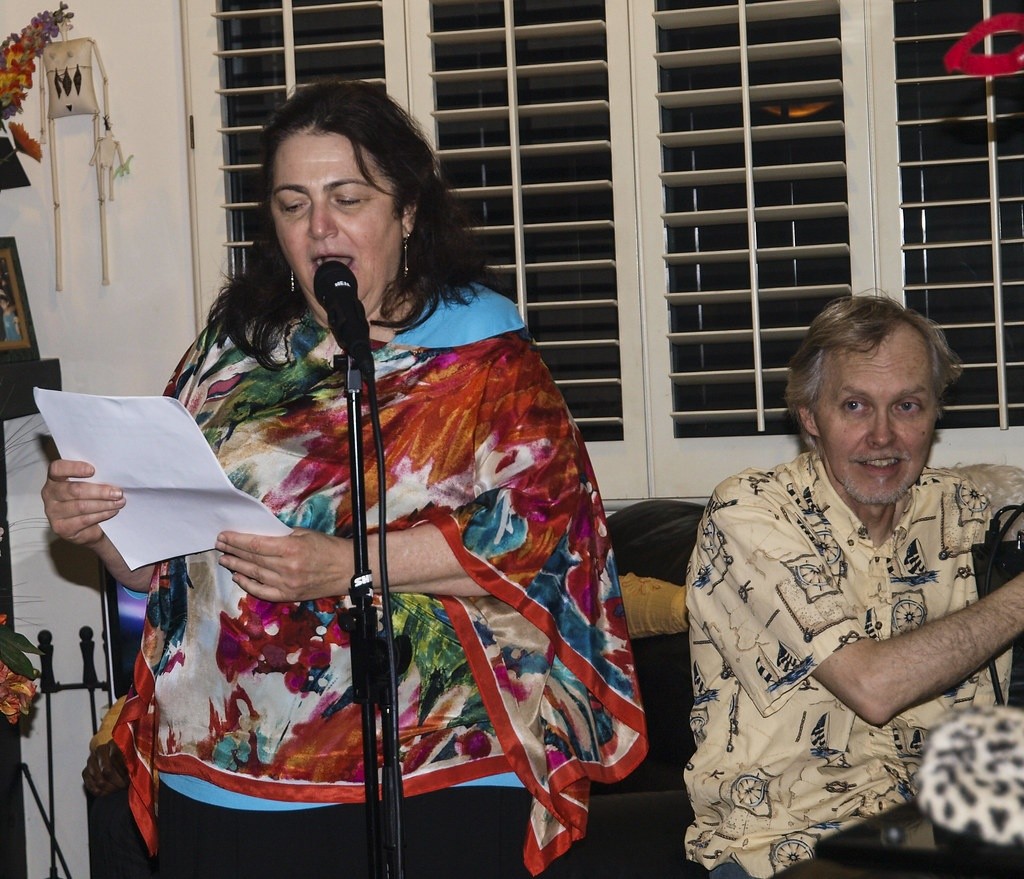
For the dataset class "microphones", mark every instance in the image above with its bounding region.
[314,260,374,373]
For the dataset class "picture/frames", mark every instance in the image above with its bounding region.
[0,236,40,364]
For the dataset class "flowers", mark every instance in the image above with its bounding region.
[0,614,46,724]
[0,3,74,163]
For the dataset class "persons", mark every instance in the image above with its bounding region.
[38,80,648,879]
[1,289,23,342]
[681,289,1024,878]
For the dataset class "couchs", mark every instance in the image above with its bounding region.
[566,500,705,879]
